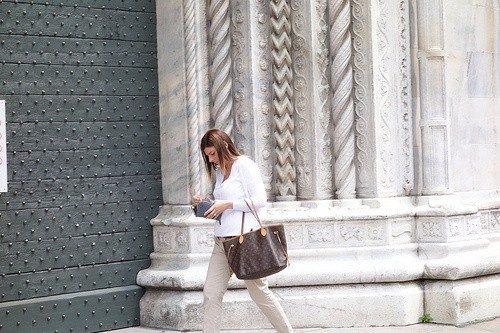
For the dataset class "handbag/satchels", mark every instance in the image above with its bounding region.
[222,198,291,279]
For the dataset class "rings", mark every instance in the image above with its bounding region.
[214,214,216,216]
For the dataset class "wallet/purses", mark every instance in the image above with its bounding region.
[191,196,221,225]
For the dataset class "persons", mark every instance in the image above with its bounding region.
[191,129,298,333]
